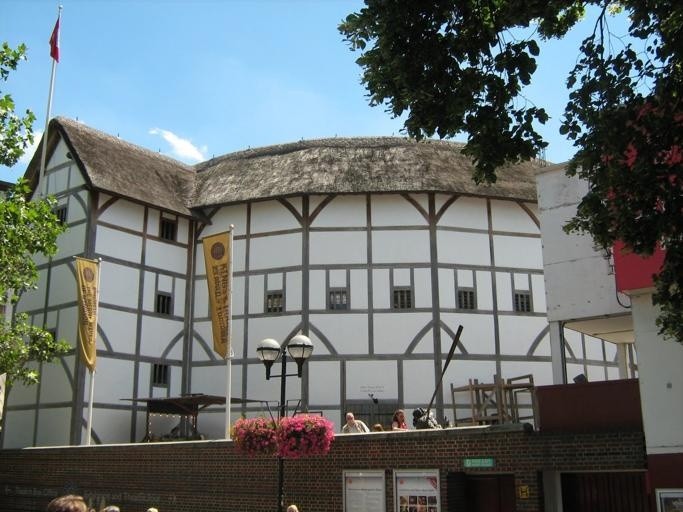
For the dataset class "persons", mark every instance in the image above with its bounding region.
[47,494,86,512]
[103,505,120,512]
[287,505,299,512]
[413,407,442,429]
[342,412,370,433]
[391,409,408,431]
[147,507,158,512]
[371,424,384,432]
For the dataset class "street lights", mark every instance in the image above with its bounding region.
[256,334,315,512]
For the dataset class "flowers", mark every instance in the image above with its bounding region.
[229,416,335,458]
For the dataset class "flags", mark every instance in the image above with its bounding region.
[48,17,60,63]
